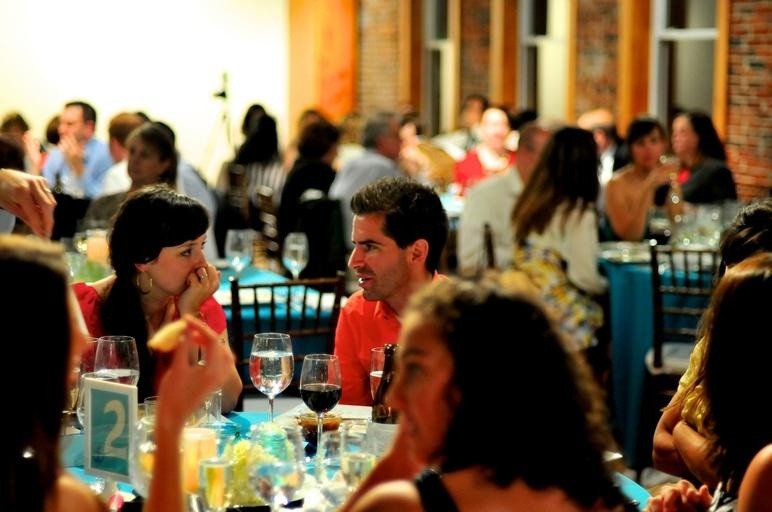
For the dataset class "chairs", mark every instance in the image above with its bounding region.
[229,272,347,411]
[643,239,720,448]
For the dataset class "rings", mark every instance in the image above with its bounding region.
[201,274,208,279]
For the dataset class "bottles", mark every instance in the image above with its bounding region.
[370,346,397,424]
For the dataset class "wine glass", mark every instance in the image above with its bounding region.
[300,353,345,466]
[249,330,294,428]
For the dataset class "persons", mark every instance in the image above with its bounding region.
[341,280,639,511]
[0,101,222,264]
[1,233,236,512]
[72,184,242,415]
[215,96,739,350]
[642,199,772,511]
[328,181,450,407]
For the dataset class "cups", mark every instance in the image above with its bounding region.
[636,199,749,254]
[280,232,309,279]
[182,389,370,512]
[47,171,86,202]
[659,156,681,180]
[59,229,110,285]
[128,416,191,503]
[58,336,158,438]
[370,346,387,400]
[225,227,255,276]
